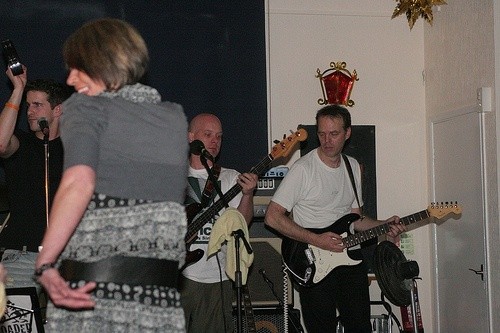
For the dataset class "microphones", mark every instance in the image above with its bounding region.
[37,116,53,137]
[260,269,269,281]
[189,140,213,161]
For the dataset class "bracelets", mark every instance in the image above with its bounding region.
[33,262,55,283]
[4,101,20,111]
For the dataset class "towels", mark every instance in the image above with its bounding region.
[206,207,255,286]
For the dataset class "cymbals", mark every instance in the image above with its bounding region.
[1,38,25,76]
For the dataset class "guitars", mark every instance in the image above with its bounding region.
[280,201,463,296]
[241,285,257,333]
[181,128,308,273]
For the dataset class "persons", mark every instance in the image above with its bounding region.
[265,106,406,333]
[176,112,259,333]
[0,17,189,333]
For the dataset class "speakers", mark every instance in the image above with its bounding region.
[231,218,301,333]
[297,125,377,221]
